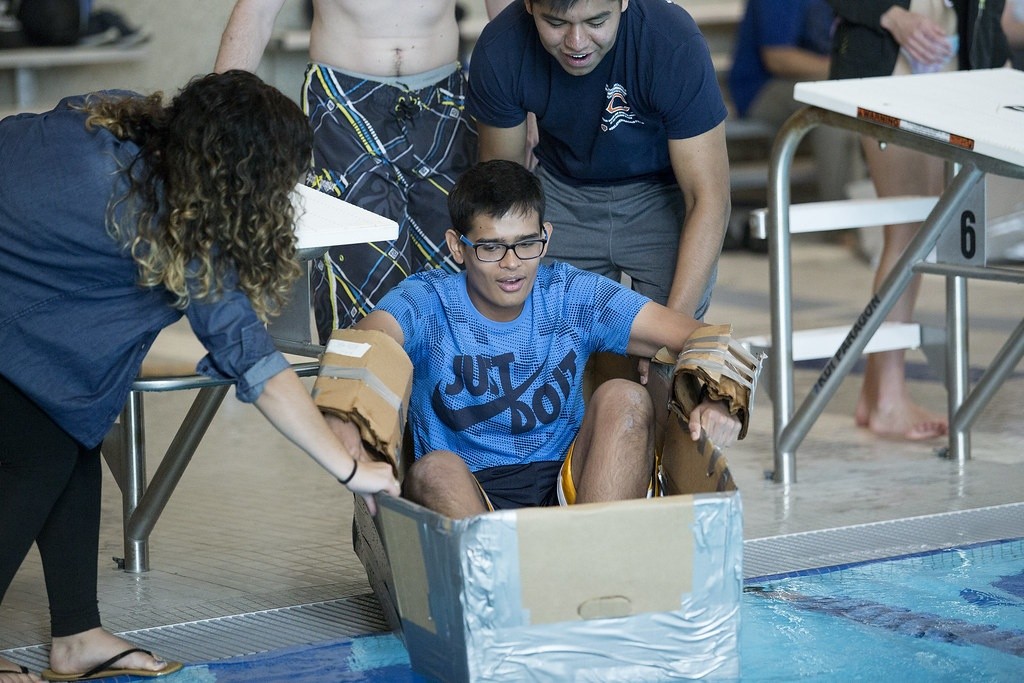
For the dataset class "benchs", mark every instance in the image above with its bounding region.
[0,41,150,116]
[267,0,873,244]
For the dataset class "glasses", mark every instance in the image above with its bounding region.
[451,221,549,262]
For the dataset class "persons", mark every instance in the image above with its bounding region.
[826,0,1024,443]
[729,0,881,247]
[323,159,745,522]
[1,69,402,682]
[212,2,517,347]
[465,0,733,387]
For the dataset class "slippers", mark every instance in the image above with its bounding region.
[0,664,35,683]
[40,647,183,680]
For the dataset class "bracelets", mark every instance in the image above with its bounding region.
[341,457,359,484]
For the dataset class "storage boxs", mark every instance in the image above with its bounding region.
[346,348,745,683]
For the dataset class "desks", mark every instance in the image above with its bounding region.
[101,181,402,580]
[750,61,1023,488]
